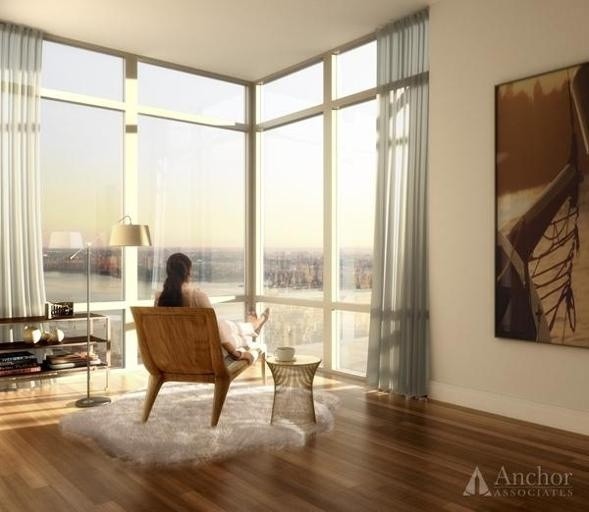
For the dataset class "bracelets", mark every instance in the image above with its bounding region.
[232,350,243,361]
[154,253,270,366]
[228,347,236,354]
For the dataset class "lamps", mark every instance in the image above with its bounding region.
[70,216,151,409]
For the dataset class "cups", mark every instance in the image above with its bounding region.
[274,348,295,361]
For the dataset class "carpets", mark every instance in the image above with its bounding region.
[60,381,339,477]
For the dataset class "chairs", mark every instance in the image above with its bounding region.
[128,304,266,427]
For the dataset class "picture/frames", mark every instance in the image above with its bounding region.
[494,62,589,349]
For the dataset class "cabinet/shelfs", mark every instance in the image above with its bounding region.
[1,314,115,401]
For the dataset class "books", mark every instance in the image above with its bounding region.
[0,351,42,377]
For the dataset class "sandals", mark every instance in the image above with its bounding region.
[247,306,270,336]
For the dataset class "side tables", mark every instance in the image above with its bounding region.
[264,358,319,425]
